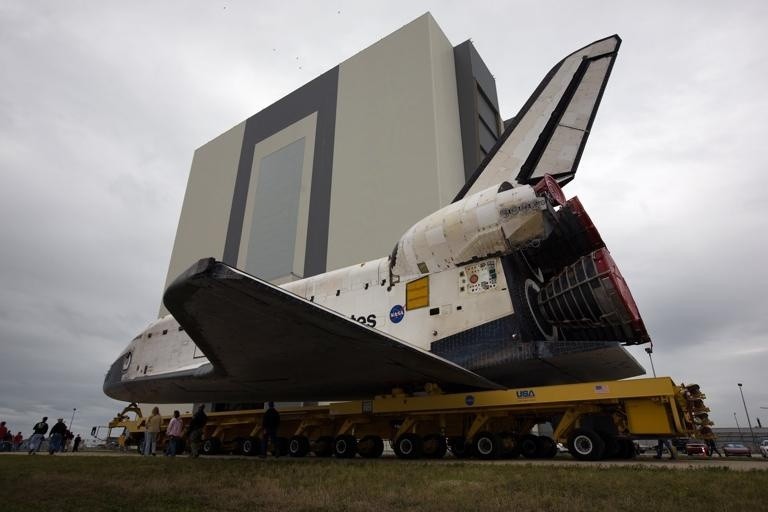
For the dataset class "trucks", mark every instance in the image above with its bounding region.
[106,376,715,460]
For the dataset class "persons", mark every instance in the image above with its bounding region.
[34,435,45,453]
[0,420,7,452]
[162,410,183,457]
[12,431,23,452]
[47,416,66,455]
[185,402,209,459]
[61,426,70,453]
[143,406,164,457]
[258,401,282,460]
[704,438,721,458]
[118,432,127,454]
[72,433,82,453]
[64,431,74,452]
[27,417,49,456]
[3,430,13,452]
[650,439,676,461]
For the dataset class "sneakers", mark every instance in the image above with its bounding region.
[652,452,677,460]
[27,448,56,455]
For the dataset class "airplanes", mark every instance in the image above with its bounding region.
[104,35,651,403]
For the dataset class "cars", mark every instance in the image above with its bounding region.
[686,444,707,455]
[760,440,768,457]
[725,442,751,458]
[556,443,569,453]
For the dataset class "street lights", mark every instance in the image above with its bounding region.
[738,383,757,450]
[645,347,657,378]
